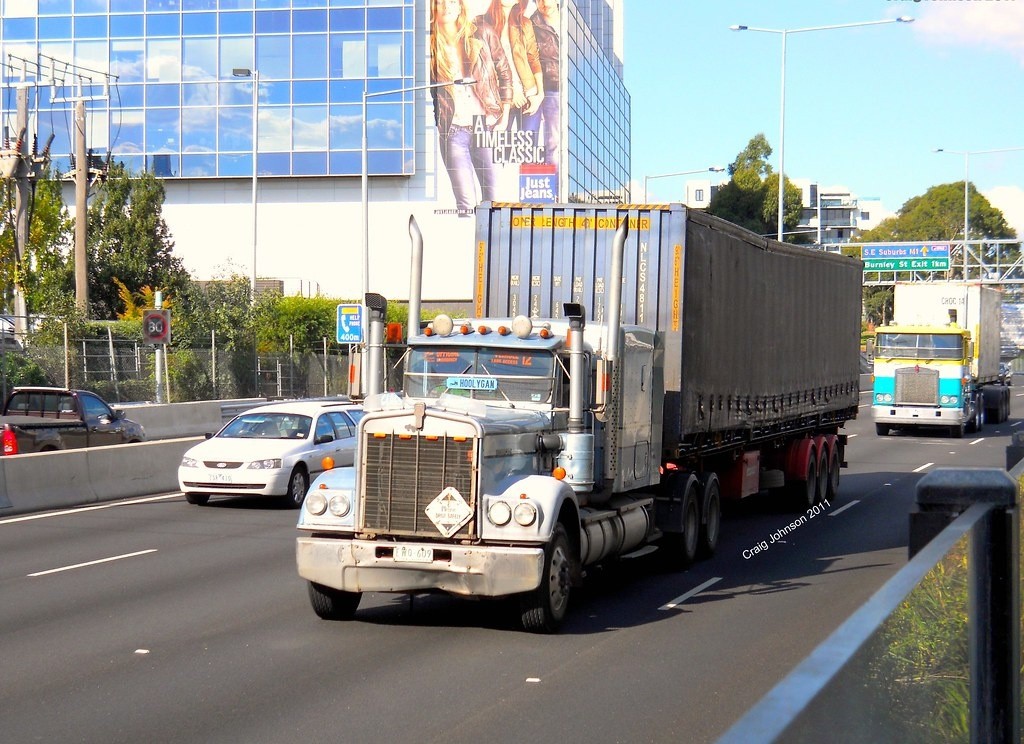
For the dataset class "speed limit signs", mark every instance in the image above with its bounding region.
[141,307,172,346]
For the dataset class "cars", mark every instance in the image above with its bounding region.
[176,395,371,511]
[0,313,39,357]
[994,361,1014,386]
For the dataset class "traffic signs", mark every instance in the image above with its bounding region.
[861,243,951,273]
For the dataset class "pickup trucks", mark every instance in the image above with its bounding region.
[0,385,149,456]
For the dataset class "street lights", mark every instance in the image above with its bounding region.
[359,75,481,397]
[933,146,1024,280]
[812,190,854,250]
[231,68,259,306]
[643,166,725,204]
[727,16,917,243]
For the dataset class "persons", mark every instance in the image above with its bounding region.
[430,360,498,400]
[429,0,560,214]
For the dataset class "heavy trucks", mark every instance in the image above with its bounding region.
[286,201,865,635]
[863,281,1011,438]
